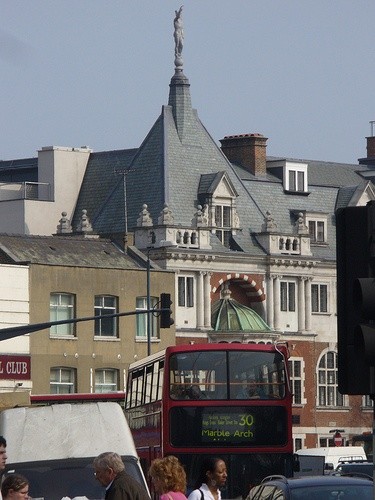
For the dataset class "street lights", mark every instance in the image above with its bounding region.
[147,241,174,356]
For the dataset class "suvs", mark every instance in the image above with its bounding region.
[244,459,375,500]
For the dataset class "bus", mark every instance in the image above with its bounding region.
[122,343,300,500]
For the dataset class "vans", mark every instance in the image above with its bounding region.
[0,401,152,500]
[293,446,367,476]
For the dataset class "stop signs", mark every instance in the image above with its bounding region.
[333,433,343,447]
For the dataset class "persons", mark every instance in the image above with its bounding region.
[149,456,188,500]
[93,453,151,500]
[0,436,30,500]
[184,385,206,400]
[235,380,259,398]
[173,4,185,58]
[187,456,228,500]
